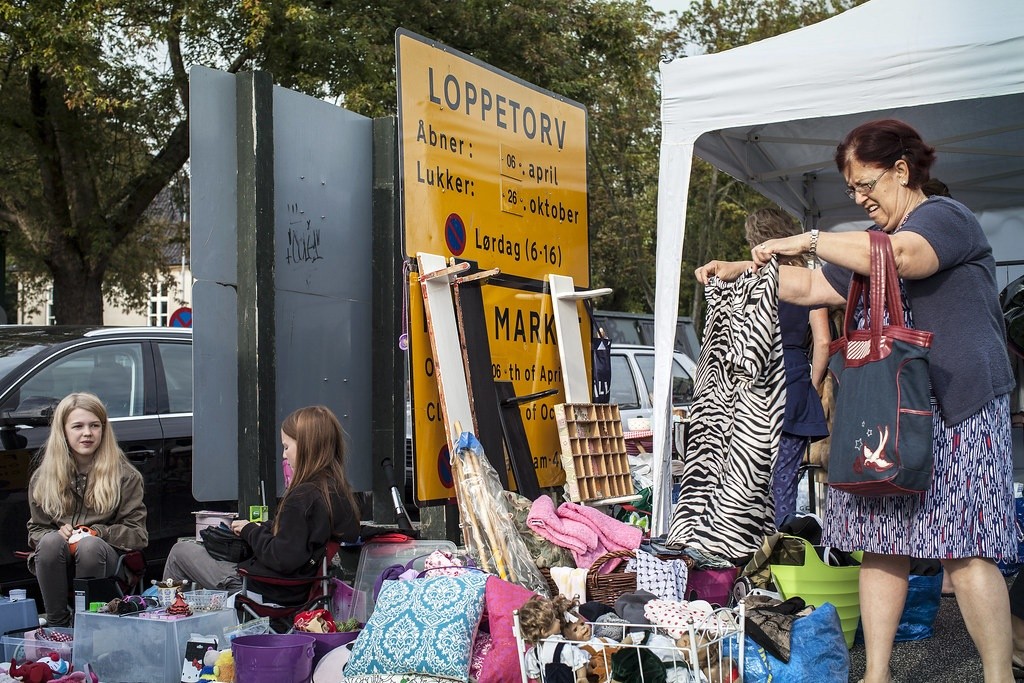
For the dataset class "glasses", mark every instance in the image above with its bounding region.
[845,160,901,201]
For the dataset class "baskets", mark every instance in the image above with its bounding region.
[587,550,639,604]
[185,590,229,613]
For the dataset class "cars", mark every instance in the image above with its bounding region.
[0,322,239,614]
[610,346,698,433]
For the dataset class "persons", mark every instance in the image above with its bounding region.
[519,593,739,683]
[694,118,1019,683]
[163,405,361,605]
[744,204,830,526]
[28,392,149,630]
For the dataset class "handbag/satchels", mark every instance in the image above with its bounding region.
[584,297,611,404]
[827,228,935,496]
[200,523,255,563]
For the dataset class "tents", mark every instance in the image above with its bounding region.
[651,0,1024,540]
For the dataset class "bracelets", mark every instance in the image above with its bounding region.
[810,229,818,256]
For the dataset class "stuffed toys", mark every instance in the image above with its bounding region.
[9,651,98,683]
[199,647,233,683]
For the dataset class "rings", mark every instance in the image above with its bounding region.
[760,243,766,249]
